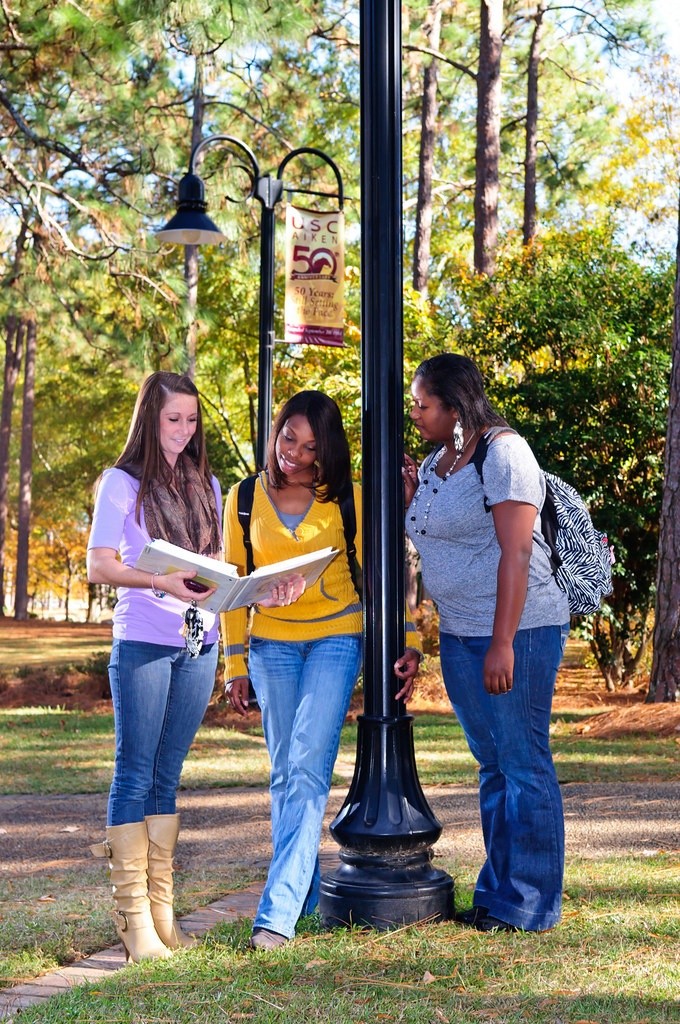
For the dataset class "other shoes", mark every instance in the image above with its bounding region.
[450,905,527,933]
[247,928,290,951]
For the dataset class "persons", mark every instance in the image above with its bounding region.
[224,389,425,950]
[401,353,570,933]
[86,373,304,963]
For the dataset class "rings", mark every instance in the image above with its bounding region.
[279,596,285,600]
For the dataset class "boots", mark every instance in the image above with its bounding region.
[88,821,176,965]
[144,813,203,950]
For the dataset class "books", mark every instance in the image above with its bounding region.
[134,539,340,614]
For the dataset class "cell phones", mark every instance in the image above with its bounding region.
[184,580,209,593]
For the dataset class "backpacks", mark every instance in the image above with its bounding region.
[472,432,616,616]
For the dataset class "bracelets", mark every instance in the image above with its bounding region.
[151,573,166,598]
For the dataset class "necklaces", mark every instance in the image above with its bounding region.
[411,432,475,535]
